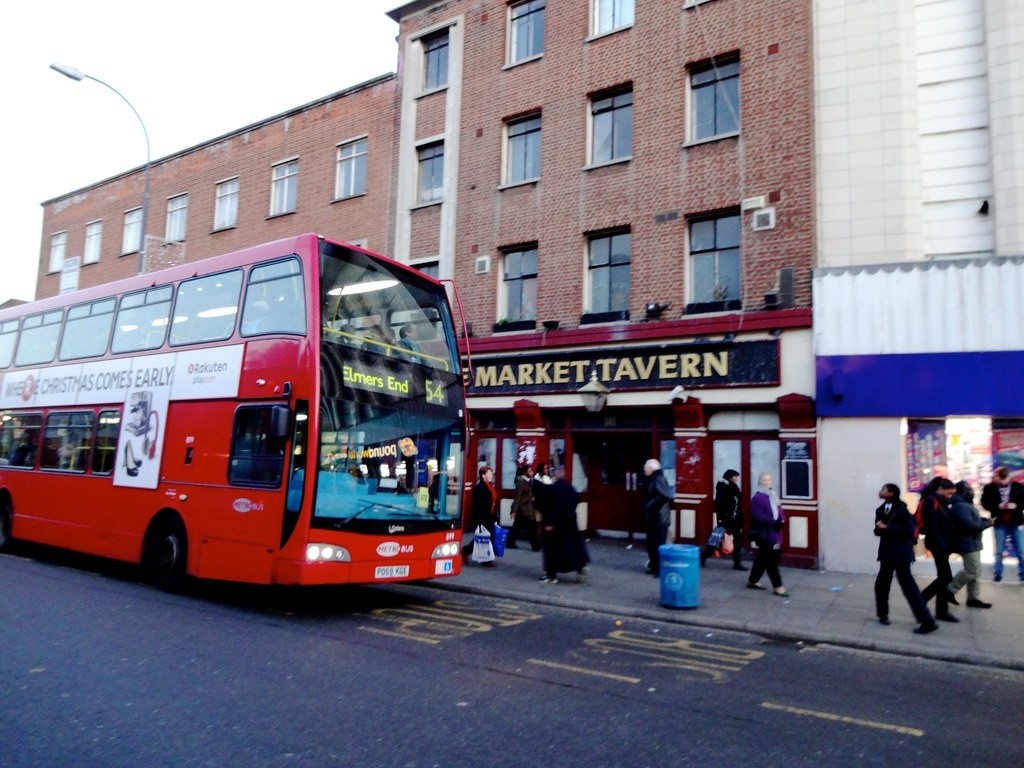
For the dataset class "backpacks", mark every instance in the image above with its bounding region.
[915,496,938,537]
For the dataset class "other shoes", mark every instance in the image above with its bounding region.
[936,612,959,622]
[482,562,496,567]
[540,576,559,584]
[645,568,658,577]
[699,552,705,567]
[733,564,748,571]
[773,590,789,597]
[967,600,991,608]
[994,574,1002,581]
[460,546,468,564]
[505,542,519,549]
[747,583,766,590]
[947,590,959,605]
[877,615,890,625]
[914,621,938,634]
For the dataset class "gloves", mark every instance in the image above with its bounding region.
[773,520,784,532]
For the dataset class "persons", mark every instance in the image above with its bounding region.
[643,459,670,576]
[504,463,591,584]
[746,472,790,596]
[337,324,356,345]
[367,325,394,354]
[12,428,40,466]
[872,484,940,634]
[948,482,994,608]
[982,467,1023,582]
[916,476,960,623]
[396,325,427,365]
[701,469,749,570]
[460,467,499,567]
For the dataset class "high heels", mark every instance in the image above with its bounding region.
[125,440,141,477]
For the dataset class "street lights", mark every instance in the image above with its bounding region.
[47,62,152,277]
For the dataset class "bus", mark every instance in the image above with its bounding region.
[0,232,474,597]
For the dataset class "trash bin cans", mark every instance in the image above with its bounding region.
[658,544,701,607]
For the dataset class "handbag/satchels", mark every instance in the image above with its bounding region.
[706,527,725,548]
[721,533,734,555]
[472,525,495,562]
[493,521,509,556]
